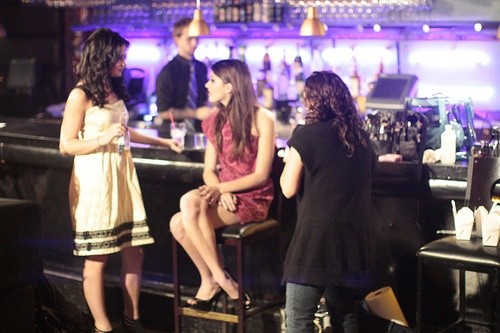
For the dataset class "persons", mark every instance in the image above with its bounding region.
[169,60,278,312]
[60,29,184,333]
[154,17,208,139]
[280,70,380,333]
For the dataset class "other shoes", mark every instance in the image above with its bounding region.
[123,313,146,333]
[92,327,117,333]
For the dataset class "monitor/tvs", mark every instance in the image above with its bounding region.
[365,73,418,111]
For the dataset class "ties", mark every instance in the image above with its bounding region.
[185,59,198,109]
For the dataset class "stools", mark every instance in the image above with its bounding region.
[172,217,282,333]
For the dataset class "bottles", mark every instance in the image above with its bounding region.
[440,124,456,166]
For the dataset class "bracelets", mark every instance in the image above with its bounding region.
[96,136,101,150]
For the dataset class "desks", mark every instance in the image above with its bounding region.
[0,196,44,303]
[416,220,500,333]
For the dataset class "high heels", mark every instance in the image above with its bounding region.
[189,288,224,311]
[227,295,247,315]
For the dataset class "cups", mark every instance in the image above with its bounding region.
[193,134,207,149]
[169,122,187,150]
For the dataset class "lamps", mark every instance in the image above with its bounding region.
[299,7,325,36]
[187,0,209,37]
